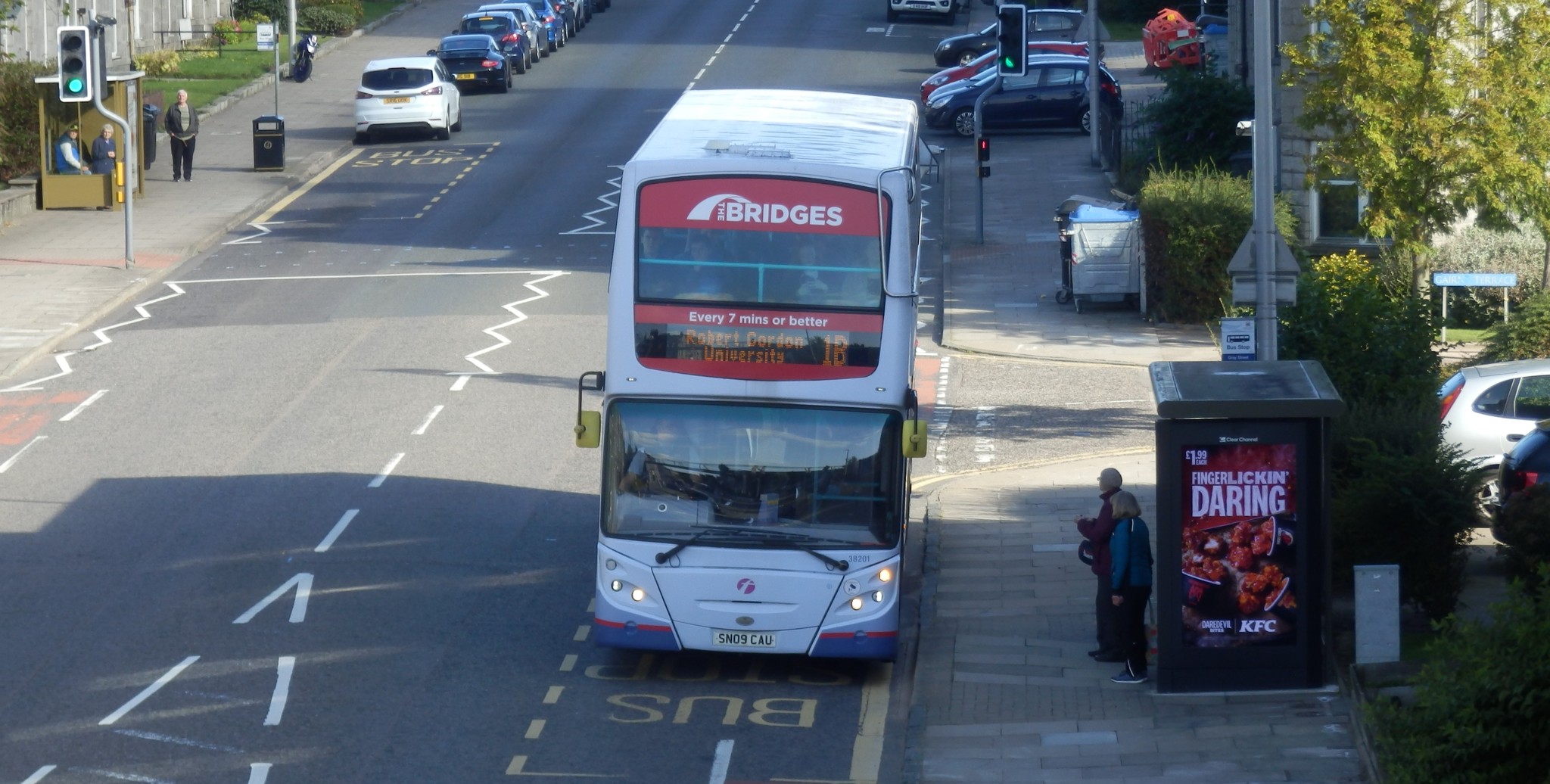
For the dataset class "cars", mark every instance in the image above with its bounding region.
[501,0,612,53]
[1491,418,1550,562]
[475,2,550,63]
[917,39,1106,105]
[353,57,462,146]
[924,53,1124,140]
[885,0,957,26]
[426,34,514,94]
[451,10,533,74]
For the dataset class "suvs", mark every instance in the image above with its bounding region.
[1435,358,1550,525]
[931,7,1089,69]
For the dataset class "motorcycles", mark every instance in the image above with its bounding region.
[292,34,319,83]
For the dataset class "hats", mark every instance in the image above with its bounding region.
[69,125,78,130]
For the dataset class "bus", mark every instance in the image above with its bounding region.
[570,87,928,668]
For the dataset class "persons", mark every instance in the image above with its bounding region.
[56,122,116,211]
[164,90,199,182]
[638,227,839,302]
[619,414,703,496]
[1108,491,1152,683]
[1073,468,1122,663]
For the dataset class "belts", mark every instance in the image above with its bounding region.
[183,140,187,147]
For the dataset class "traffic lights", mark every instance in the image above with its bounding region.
[997,3,1028,77]
[977,137,990,162]
[995,6,1027,79]
[56,25,91,102]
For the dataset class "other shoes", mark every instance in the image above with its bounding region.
[1141,671,1148,680]
[185,178,192,181]
[173,178,179,182]
[1088,650,1104,657]
[103,206,112,209]
[1095,652,1115,662]
[1111,672,1144,683]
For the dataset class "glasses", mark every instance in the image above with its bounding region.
[1097,477,1101,481]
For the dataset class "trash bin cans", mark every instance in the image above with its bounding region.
[253,116,285,172]
[143,112,154,170]
[143,104,161,162]
[1055,195,1126,305]
[1066,205,1147,314]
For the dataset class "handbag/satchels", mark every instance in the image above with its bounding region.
[1144,598,1159,665]
[1078,540,1095,565]
